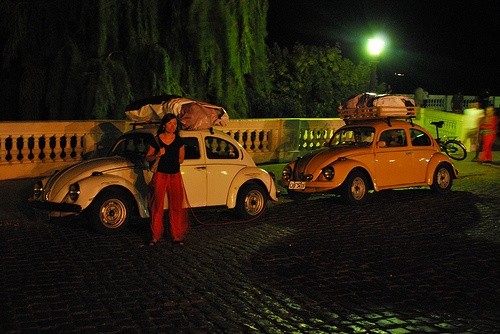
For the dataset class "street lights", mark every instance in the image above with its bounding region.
[366,37,386,93]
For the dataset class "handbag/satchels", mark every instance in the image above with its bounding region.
[142,170,154,192]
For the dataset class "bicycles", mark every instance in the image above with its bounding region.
[430,121,467,161]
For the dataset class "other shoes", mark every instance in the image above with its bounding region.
[178,240,185,247]
[149,239,158,247]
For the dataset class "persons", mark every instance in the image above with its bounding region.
[462,102,497,165]
[385,129,403,147]
[146,113,186,246]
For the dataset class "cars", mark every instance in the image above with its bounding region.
[277,116,459,207]
[27,121,281,236]
